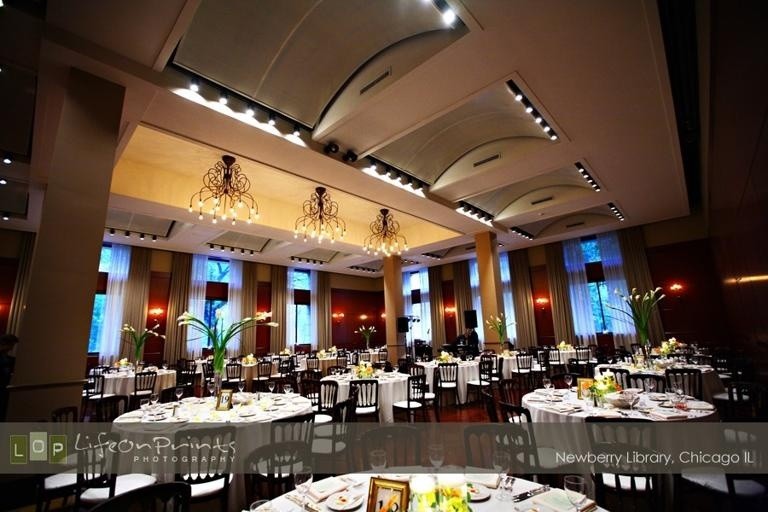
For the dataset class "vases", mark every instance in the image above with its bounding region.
[366,336,370,349]
[213,351,223,396]
[499,336,505,354]
[134,348,140,374]
[638,329,647,346]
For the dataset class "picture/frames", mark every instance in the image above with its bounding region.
[577,378,593,400]
[366,476,410,512]
[634,355,645,368]
[216,390,233,411]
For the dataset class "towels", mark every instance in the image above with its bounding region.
[464,466,503,488]
[551,403,576,414]
[650,408,689,422]
[534,486,587,512]
[308,476,346,500]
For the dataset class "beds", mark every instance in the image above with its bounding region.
[250,465,611,512]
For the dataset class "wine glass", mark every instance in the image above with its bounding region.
[564,476,589,512]
[624,391,639,419]
[645,377,656,399]
[284,385,290,398]
[544,379,551,397]
[286,388,293,401]
[371,450,386,478]
[466,355,473,364]
[268,382,275,395]
[492,450,512,501]
[607,356,613,366]
[140,398,149,421]
[294,466,313,512]
[580,389,592,413]
[162,362,168,370]
[393,365,400,375]
[207,383,215,398]
[428,443,444,474]
[332,367,346,378]
[249,500,272,512]
[150,391,159,416]
[564,375,572,396]
[176,388,182,406]
[381,364,385,370]
[417,356,420,363]
[238,382,244,395]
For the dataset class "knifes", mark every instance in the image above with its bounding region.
[284,494,318,512]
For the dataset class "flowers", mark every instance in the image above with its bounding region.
[351,363,373,379]
[654,337,679,355]
[115,357,129,367]
[328,345,337,353]
[245,353,253,364]
[280,347,290,355]
[415,483,474,512]
[117,323,166,359]
[353,325,377,340]
[583,376,622,399]
[178,308,279,372]
[591,286,665,343]
[436,351,453,364]
[317,349,326,358]
[485,312,517,344]
[556,340,569,350]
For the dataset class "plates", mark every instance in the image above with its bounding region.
[274,401,287,404]
[264,406,278,411]
[163,403,177,409]
[147,415,166,420]
[148,410,165,415]
[326,493,363,511]
[462,482,490,500]
[238,411,257,417]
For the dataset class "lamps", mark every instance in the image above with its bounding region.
[363,208,408,258]
[294,186,346,244]
[188,154,259,226]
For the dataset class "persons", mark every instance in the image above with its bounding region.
[0,331,18,416]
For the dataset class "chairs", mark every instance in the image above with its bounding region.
[312,399,353,471]
[94,367,109,375]
[244,440,308,510]
[682,421,768,512]
[438,362,461,409]
[630,343,640,354]
[350,379,379,423]
[466,360,493,410]
[327,366,346,376]
[481,388,499,426]
[510,353,532,390]
[301,370,321,393]
[599,367,629,388]
[392,374,426,424]
[712,365,760,416]
[588,344,598,366]
[360,352,370,362]
[626,374,666,394]
[546,348,565,379]
[584,416,657,454]
[298,378,320,413]
[337,355,347,367]
[567,347,589,377]
[409,364,425,394]
[345,424,421,473]
[251,361,272,393]
[270,412,315,473]
[81,375,116,424]
[463,422,530,482]
[156,422,234,512]
[727,383,768,423]
[202,363,214,397]
[272,358,281,364]
[665,368,703,402]
[128,371,157,412]
[161,385,187,405]
[498,401,573,487]
[90,481,191,512]
[536,348,546,368]
[306,357,319,370]
[182,360,195,376]
[379,351,387,361]
[94,395,128,439]
[480,354,504,398]
[304,380,342,428]
[408,367,441,423]
[592,440,674,512]
[50,406,78,433]
[41,431,120,512]
[222,363,242,391]
